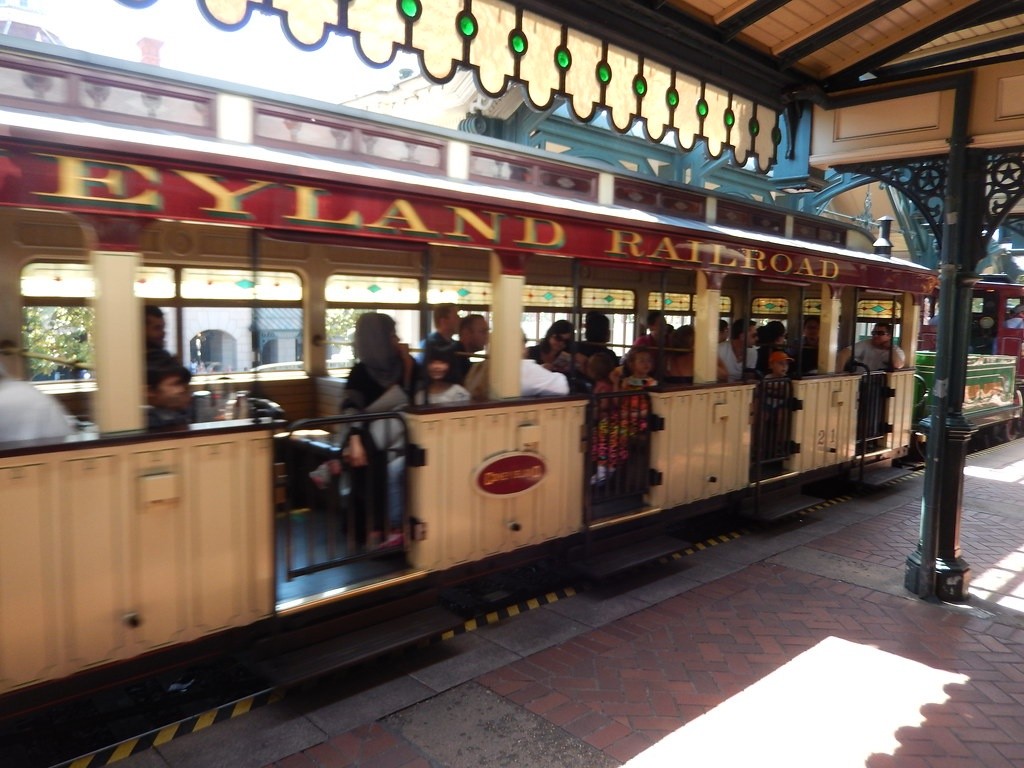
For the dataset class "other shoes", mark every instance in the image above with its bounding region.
[308,460,335,491]
[381,529,404,547]
[589,476,608,491]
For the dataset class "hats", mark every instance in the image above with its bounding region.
[768,350,794,363]
[1009,304,1024,313]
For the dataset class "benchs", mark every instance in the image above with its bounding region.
[277,423,344,456]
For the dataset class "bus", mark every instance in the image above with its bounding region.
[0,37,1024,693]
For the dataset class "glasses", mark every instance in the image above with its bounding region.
[550,334,570,343]
[871,331,888,336]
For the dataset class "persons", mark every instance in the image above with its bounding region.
[992,305,1024,355]
[1,298,905,550]
